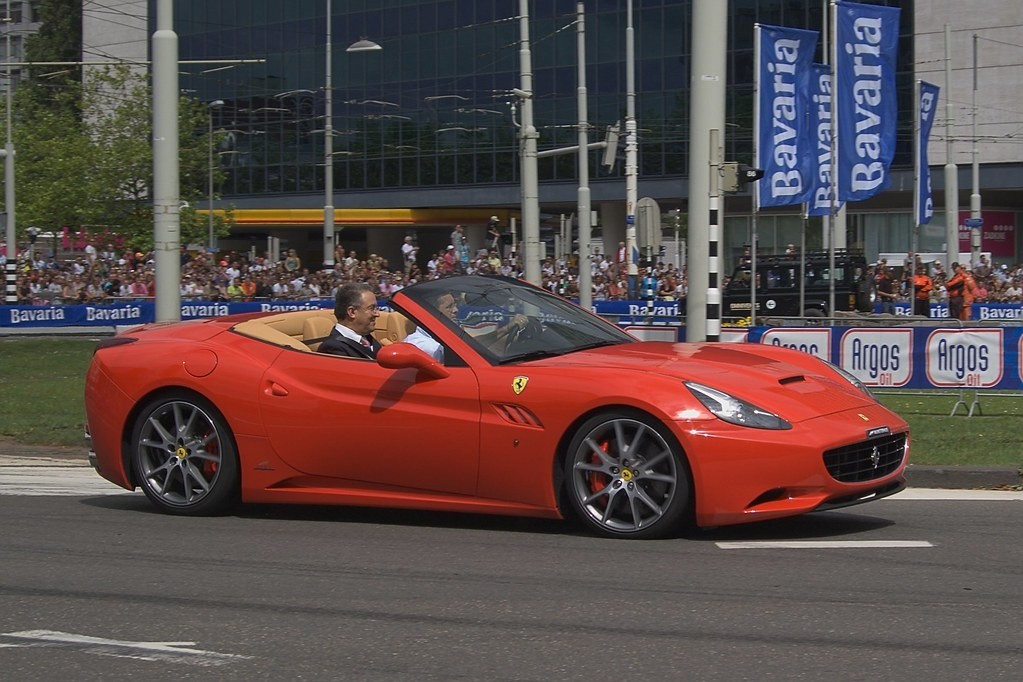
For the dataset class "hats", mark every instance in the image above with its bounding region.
[951,262,958,268]
[1000,264,1008,269]
[447,245,454,249]
[491,216,500,221]
[135,252,144,257]
[220,261,228,266]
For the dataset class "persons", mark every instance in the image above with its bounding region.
[2,216,1023,325]
[402,291,529,367]
[312,283,383,362]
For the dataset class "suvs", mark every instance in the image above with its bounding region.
[719,247,875,324]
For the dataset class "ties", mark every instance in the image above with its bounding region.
[360,338,371,349]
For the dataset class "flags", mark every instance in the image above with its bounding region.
[836,0,900,202]
[801,63,846,219]
[919,79,941,224]
[759,23,820,208]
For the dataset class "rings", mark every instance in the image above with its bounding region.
[520,320,523,323]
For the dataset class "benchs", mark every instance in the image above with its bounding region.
[254,310,342,346]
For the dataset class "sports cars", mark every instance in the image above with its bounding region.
[83,272,912,540]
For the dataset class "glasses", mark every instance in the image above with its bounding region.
[353,305,381,311]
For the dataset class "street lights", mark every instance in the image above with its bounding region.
[207,101,225,265]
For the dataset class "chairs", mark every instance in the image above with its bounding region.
[303,317,335,352]
[379,311,417,345]
